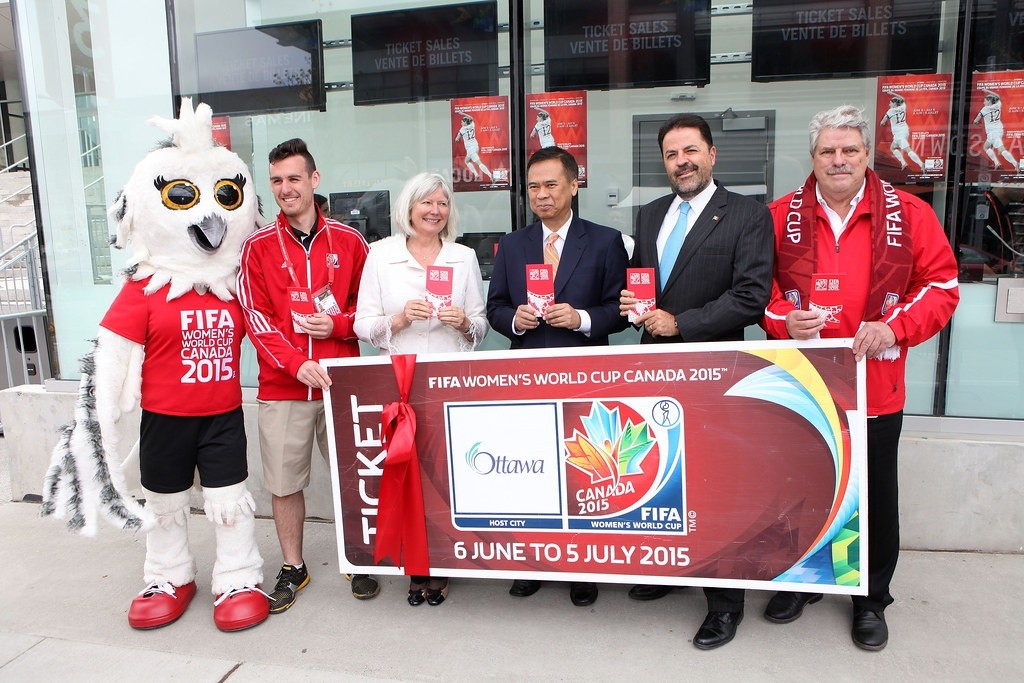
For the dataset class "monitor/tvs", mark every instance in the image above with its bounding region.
[971,0,1024,72]
[330,190,391,244]
[751,0,942,82]
[193,19,326,118]
[351,0,500,107]
[956,181,1024,283]
[462,232,507,280]
[544,0,712,92]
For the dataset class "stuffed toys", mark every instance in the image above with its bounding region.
[39,96,271,633]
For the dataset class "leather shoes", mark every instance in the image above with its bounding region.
[693,607,745,649]
[764,591,824,623]
[629,585,672,600]
[569,582,598,605]
[852,602,889,650]
[508,579,541,597]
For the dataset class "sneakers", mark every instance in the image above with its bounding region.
[267,559,310,613]
[345,574,380,599]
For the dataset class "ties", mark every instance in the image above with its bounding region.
[544,233,559,283]
[659,202,691,293]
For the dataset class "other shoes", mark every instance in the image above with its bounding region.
[1015,163,1022,174]
[922,163,927,175]
[901,162,908,172]
[426,577,449,605]
[994,163,1002,170]
[473,175,480,182]
[408,576,427,606]
[490,175,495,184]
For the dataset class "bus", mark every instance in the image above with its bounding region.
[892,181,1015,274]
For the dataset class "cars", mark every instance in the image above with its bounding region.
[958,245,996,283]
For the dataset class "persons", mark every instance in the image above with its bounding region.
[630,112,778,652]
[973,85,1020,173]
[760,105,962,652]
[880,91,927,176]
[232,138,379,613]
[352,171,491,606]
[485,145,633,606]
[529,106,555,148]
[455,109,494,183]
